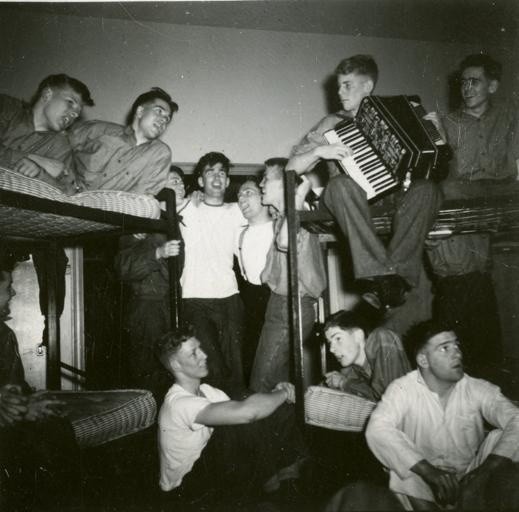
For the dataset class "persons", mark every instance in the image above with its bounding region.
[286,53,519,309]
[0,256,66,512]
[364,325,519,511]
[0,75,177,195]
[323,311,412,511]
[157,332,295,511]
[103,149,325,490]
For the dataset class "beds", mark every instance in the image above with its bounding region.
[1,165,184,452]
[278,170,517,464]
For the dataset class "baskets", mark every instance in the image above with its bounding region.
[304,384,376,434]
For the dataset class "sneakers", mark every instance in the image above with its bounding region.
[360,289,382,317]
[377,275,410,320]
